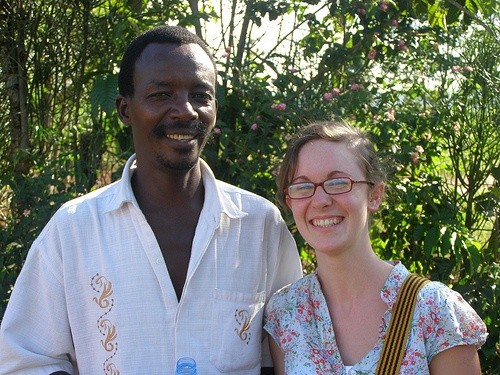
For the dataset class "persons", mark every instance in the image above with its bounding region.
[261,120,490,375]
[0,27,305,375]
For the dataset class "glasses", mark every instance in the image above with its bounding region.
[284,177,374,200]
